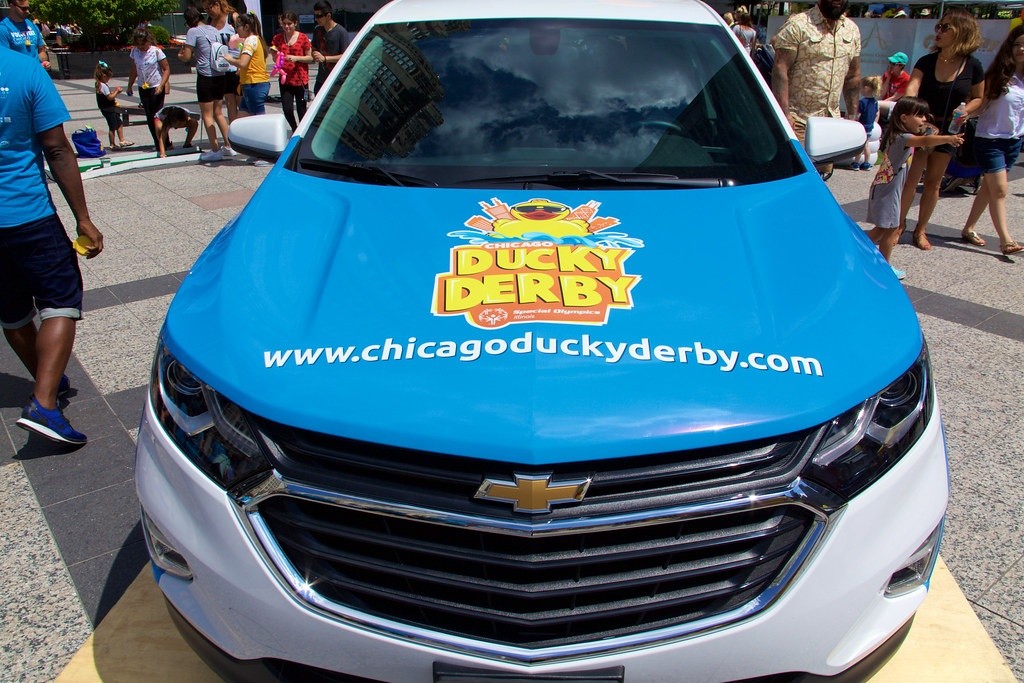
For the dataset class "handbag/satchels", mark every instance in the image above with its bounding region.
[72,127,106,158]
[156,49,170,94]
[304,90,315,101]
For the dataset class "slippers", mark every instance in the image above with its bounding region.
[1001,241,1024,255]
[962,231,985,246]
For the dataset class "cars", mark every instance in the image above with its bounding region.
[134,0,952,683]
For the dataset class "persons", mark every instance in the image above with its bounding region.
[722,0,1024,280]
[33,15,70,80]
[310,0,350,98]
[126,28,174,152]
[153,105,199,159]
[270,11,313,133]
[0,0,47,70]
[178,0,270,167]
[137,18,149,30]
[0,46,104,445]
[94,61,135,150]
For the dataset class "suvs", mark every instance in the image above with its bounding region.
[44,32,57,45]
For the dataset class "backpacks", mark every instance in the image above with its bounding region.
[194,27,231,72]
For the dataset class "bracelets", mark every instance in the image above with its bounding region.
[844,113,862,119]
[324,56,326,63]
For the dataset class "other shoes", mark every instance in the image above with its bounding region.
[166,141,174,150]
[847,162,871,170]
[201,149,221,161]
[245,157,274,167]
[182,143,193,148]
[889,263,906,280]
[152,146,156,150]
[221,145,237,156]
[109,145,124,151]
[119,140,134,147]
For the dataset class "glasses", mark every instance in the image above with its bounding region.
[16,5,28,12]
[934,24,953,32]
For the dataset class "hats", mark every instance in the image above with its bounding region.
[892,10,907,18]
[920,9,931,15]
[888,52,908,65]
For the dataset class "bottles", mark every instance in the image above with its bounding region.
[948,102,968,135]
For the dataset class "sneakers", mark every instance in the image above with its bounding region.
[56,374,71,395]
[15,397,88,445]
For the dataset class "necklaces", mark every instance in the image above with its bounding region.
[1015,71,1024,74]
[939,54,959,64]
